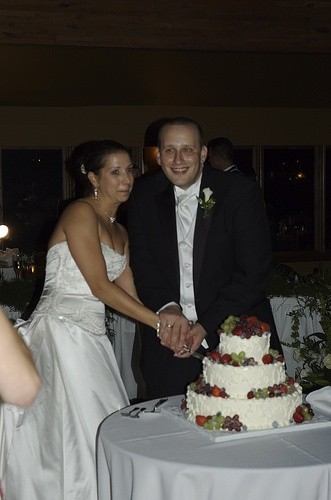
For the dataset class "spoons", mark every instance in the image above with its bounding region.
[121,406,140,416]
[144,398,168,413]
[129,407,146,418]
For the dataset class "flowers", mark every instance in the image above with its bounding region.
[267,265,331,393]
[196,187,217,220]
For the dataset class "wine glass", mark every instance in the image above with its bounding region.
[14,254,21,281]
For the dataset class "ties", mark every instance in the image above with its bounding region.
[177,196,193,238]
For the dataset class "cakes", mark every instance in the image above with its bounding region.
[182,315,315,431]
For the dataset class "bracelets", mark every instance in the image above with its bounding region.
[157,320,160,337]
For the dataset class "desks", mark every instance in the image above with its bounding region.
[95,394,330,500]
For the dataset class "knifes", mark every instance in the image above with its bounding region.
[182,345,204,360]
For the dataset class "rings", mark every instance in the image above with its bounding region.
[167,324,173,328]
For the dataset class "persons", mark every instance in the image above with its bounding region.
[122,117,271,400]
[0,139,191,500]
[207,137,242,173]
